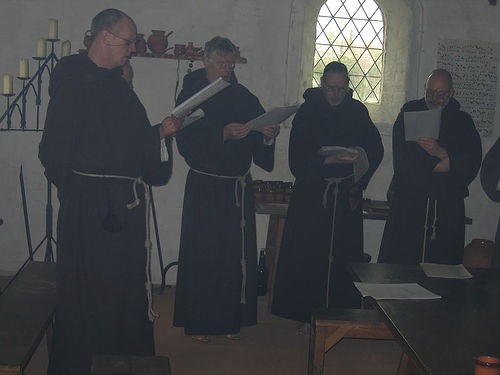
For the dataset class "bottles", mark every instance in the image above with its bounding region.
[256,250,269,297]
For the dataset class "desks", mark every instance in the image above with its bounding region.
[252,188,473,311]
[347,260,499,375]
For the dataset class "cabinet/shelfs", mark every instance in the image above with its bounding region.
[80,46,248,63]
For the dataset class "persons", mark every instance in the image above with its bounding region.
[34,7,182,374]
[173,34,282,345]
[271,59,385,325]
[377,67,483,267]
[479,127,499,274]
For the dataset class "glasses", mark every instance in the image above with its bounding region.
[323,82,348,93]
[108,31,138,46]
[207,59,236,69]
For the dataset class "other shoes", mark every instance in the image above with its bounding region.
[224,333,242,340]
[191,334,211,344]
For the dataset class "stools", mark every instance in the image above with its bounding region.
[308,308,400,375]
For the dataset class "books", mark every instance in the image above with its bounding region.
[169,76,233,119]
[352,280,442,299]
[417,260,473,280]
[400,106,445,145]
[244,97,306,137]
[317,144,371,187]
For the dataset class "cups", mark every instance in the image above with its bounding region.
[254,180,292,202]
[174,44,185,56]
[362,197,371,210]
[472,355,500,375]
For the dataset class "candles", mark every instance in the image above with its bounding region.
[36,38,47,57]
[48,16,58,39]
[2,75,13,94]
[62,40,71,56]
[19,58,30,77]
